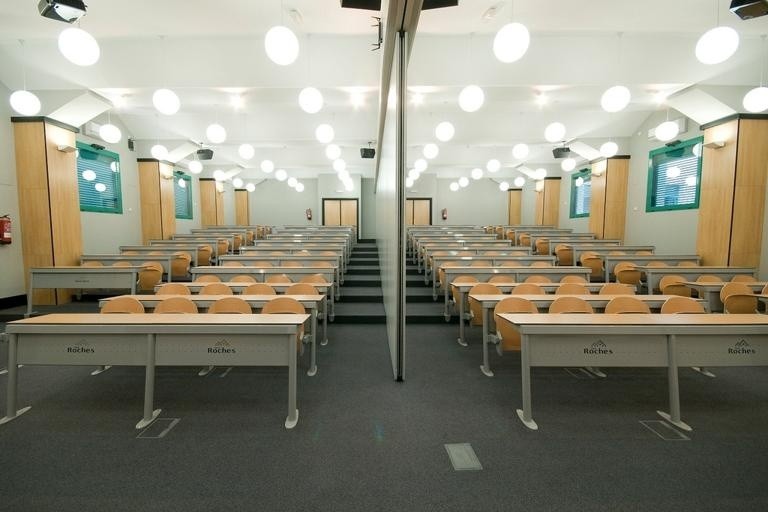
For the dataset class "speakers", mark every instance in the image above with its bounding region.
[553,148,572,159]
[197,150,213,160]
[38,0,86,24]
[361,148,375,158]
[729,0,768,21]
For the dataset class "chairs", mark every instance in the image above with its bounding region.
[468,294,768,432]
[0,225,357,431]
[403,223,768,347]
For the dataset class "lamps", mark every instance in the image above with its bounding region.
[264,24,300,66]
[10,87,357,197]
[492,23,531,63]
[694,26,741,66]
[57,28,101,69]
[405,84,767,194]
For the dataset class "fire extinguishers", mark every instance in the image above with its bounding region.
[306,208,311,220]
[0,214,12,244]
[442,208,447,220]
